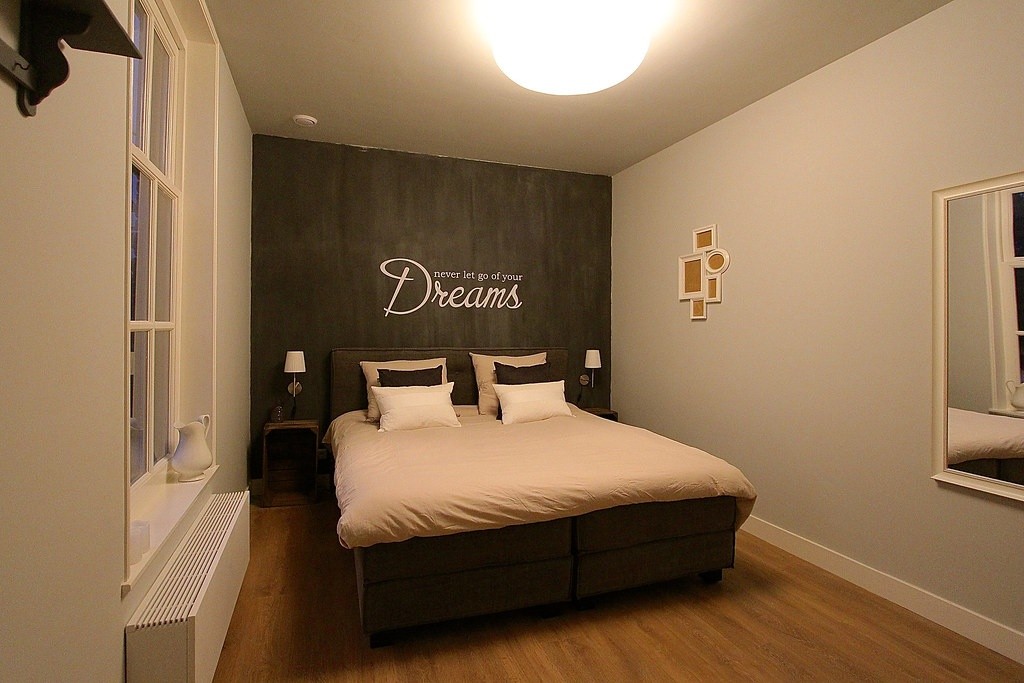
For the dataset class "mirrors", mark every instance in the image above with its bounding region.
[930,171,1024,504]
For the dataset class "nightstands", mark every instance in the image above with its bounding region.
[262,420,319,507]
[583,408,618,422]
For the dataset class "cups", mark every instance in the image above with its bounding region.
[131,520,150,554]
[129,528,142,565]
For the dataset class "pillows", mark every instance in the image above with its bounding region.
[494,362,551,420]
[360,358,447,423]
[471,352,547,415]
[378,365,443,387]
[372,382,462,432]
[492,380,576,425]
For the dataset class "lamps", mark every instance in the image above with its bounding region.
[285,351,306,398]
[580,350,601,389]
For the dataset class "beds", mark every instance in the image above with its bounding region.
[948,407,1024,481]
[321,348,757,649]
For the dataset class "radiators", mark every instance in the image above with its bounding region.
[125,491,250,683]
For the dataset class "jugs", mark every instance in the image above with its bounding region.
[1006,380,1024,411]
[172,414,213,482]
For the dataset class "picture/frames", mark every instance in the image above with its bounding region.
[678,224,729,319]
